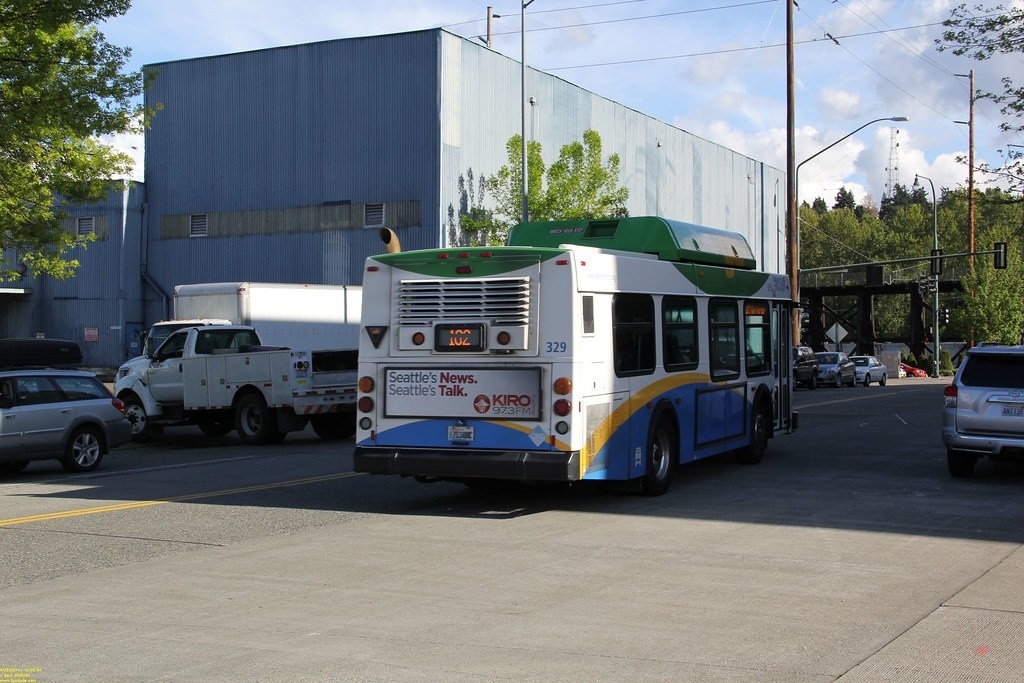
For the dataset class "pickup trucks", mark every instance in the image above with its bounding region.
[114,325,358,445]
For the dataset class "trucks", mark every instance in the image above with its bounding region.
[142,278,363,350]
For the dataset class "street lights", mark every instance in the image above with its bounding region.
[912,175,942,378]
[796,115,910,267]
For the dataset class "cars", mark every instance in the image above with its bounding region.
[0,368,132,473]
[792,346,819,391]
[814,350,857,388]
[849,355,888,388]
[900,362,929,378]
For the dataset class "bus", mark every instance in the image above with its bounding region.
[349,215,799,499]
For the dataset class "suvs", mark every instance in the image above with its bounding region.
[941,342,1024,477]
